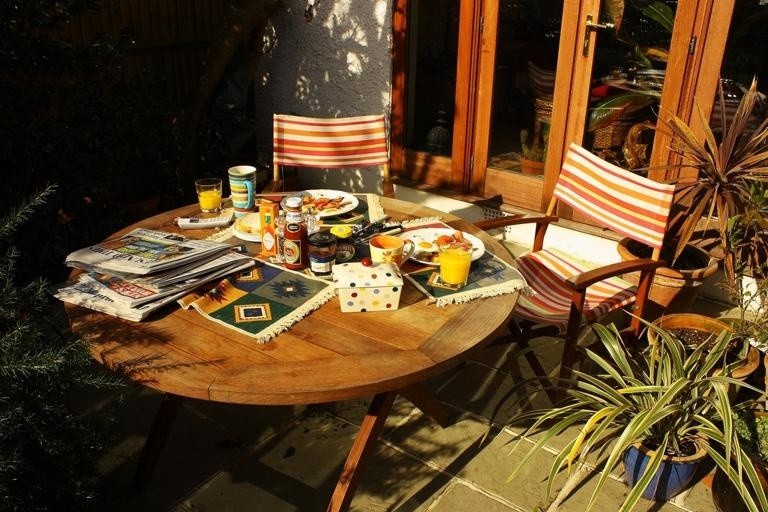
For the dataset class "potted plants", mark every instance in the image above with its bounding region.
[501,308,767,512]
[711,411,768,512]
[519,121,548,176]
[718,174,768,458]
[616,75,767,347]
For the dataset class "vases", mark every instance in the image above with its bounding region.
[646,312,760,403]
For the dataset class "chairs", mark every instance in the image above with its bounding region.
[472,142,676,409]
[272,112,395,198]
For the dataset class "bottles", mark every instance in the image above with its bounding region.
[307,232,337,276]
[262,213,274,254]
[283,197,308,270]
[330,225,360,265]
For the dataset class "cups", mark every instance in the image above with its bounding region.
[228,166,257,210]
[439,242,474,288]
[196,178,222,213]
[369,235,415,270]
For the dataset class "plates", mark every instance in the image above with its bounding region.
[280,189,359,217]
[397,228,485,266]
[233,212,322,242]
